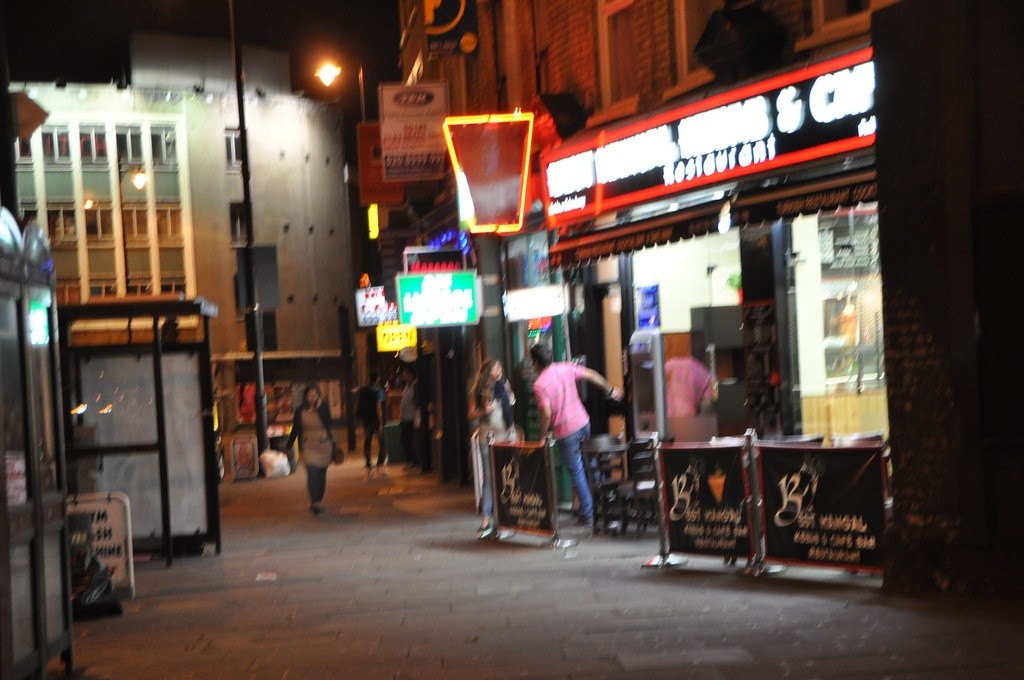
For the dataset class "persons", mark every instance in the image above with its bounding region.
[398,369,422,467]
[469,359,516,541]
[529,344,624,527]
[666,330,717,419]
[355,372,387,468]
[283,385,334,514]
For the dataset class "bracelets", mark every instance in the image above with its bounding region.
[610,386,614,395]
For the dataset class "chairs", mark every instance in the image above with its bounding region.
[575,433,658,538]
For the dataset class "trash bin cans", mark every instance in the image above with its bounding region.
[384,421,405,463]
[269,435,296,472]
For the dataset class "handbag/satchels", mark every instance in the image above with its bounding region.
[333,447,344,464]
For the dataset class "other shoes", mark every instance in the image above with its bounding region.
[310,504,321,514]
[571,517,593,527]
[476,525,491,539]
